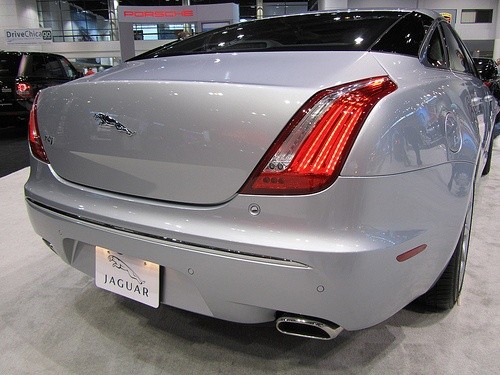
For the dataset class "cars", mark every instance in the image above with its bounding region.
[24,6,500,340]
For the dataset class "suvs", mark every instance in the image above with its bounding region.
[0,50,84,130]
